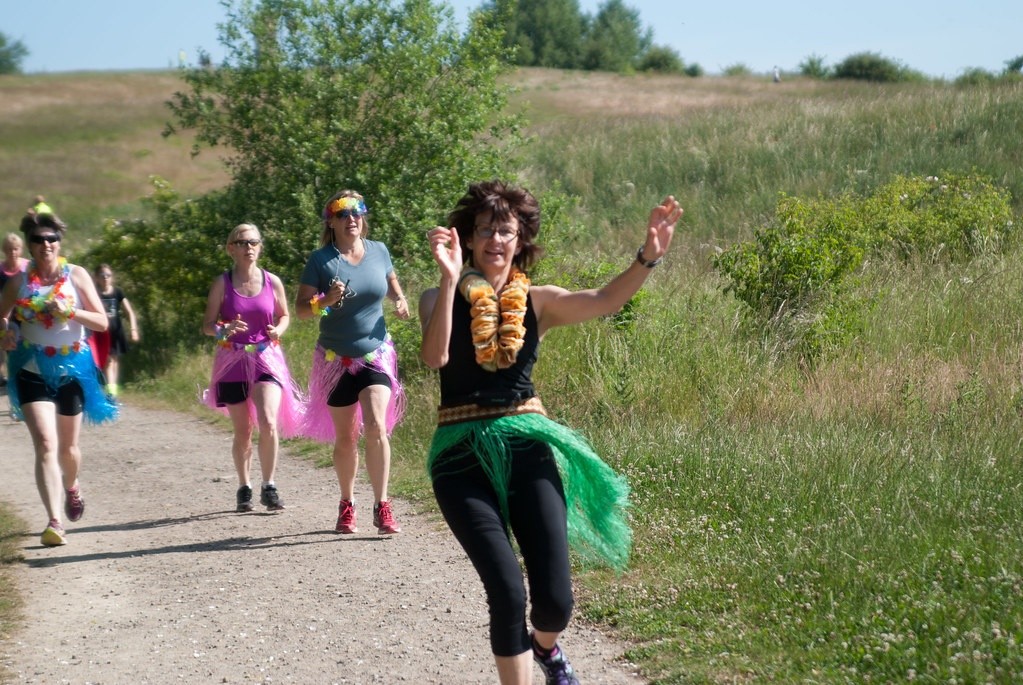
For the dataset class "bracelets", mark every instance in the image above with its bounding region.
[131,329,134,331]
[310,293,328,316]
[66,306,76,319]
[637,245,662,268]
[214,321,227,342]
[0,329,16,339]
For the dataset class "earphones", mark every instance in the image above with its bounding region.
[330,223,334,230]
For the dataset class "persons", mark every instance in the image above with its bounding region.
[418,179,683,685]
[95,263,139,397]
[0,195,121,546]
[295,190,410,535]
[203,224,307,513]
[0,233,31,385]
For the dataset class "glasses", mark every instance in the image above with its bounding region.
[331,208,362,220]
[29,235,59,244]
[472,224,520,240]
[231,238,261,246]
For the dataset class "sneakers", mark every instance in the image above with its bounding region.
[529,629,580,685]
[236,485,253,511]
[65,485,84,522]
[373,501,401,535]
[40,519,67,545]
[260,484,286,511]
[336,500,358,533]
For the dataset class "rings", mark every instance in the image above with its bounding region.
[430,241,435,247]
